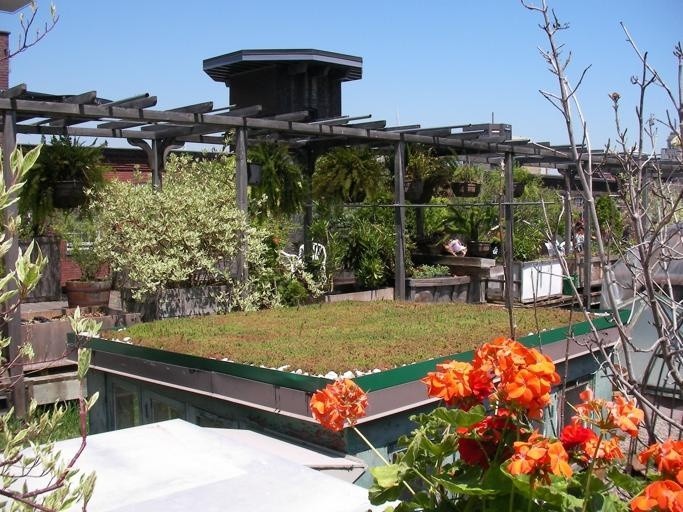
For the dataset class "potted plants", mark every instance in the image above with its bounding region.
[17,132,110,225]
[450,161,483,198]
[312,148,385,204]
[60,234,112,310]
[246,136,309,224]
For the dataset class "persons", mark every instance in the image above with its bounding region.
[428,230,466,262]
[545,221,599,256]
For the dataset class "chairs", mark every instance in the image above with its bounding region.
[275,250,298,272]
[298,241,326,284]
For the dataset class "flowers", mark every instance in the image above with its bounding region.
[307,335,683,511]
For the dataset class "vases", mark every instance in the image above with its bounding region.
[468,240,492,259]
